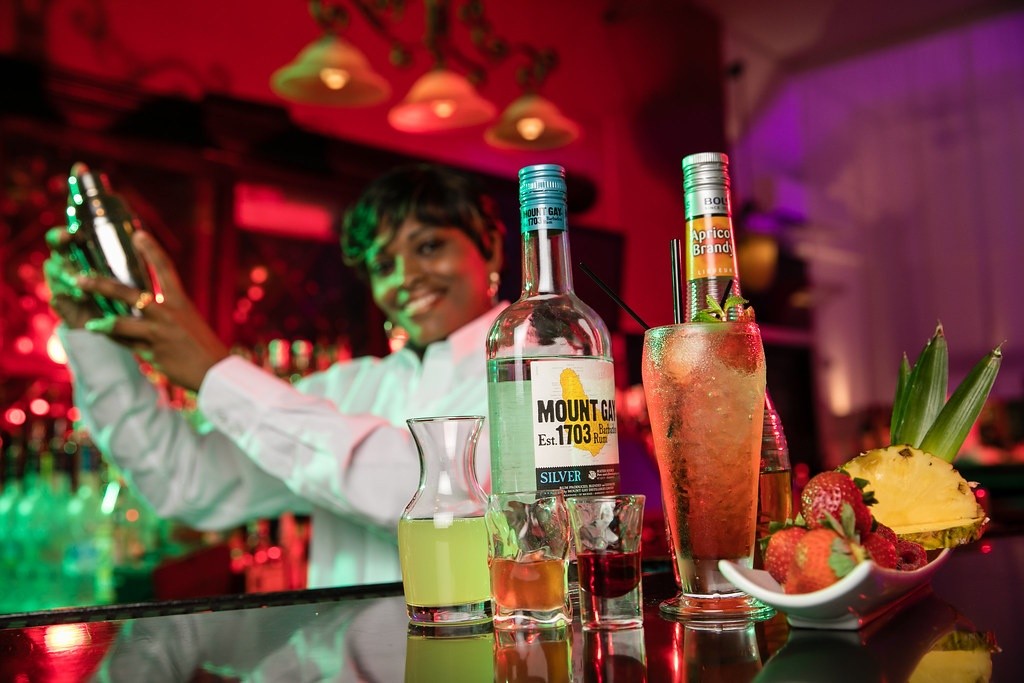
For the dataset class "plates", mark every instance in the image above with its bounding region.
[717,547,954,631]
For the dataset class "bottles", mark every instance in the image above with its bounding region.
[0,435,161,612]
[486,164,620,623]
[681,152,794,620]
[397,415,498,639]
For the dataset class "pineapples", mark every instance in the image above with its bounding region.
[834,442,986,552]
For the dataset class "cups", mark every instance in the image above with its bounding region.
[640,319,781,623]
[567,496,646,630]
[482,491,572,643]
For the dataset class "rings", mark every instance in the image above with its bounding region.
[135,291,152,309]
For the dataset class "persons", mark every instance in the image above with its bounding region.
[89,596,584,683]
[44,163,584,590]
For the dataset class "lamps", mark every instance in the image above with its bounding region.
[267,9,393,108]
[488,70,586,150]
[384,50,500,131]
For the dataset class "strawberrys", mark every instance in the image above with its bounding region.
[759,469,899,595]
[692,296,762,373]
